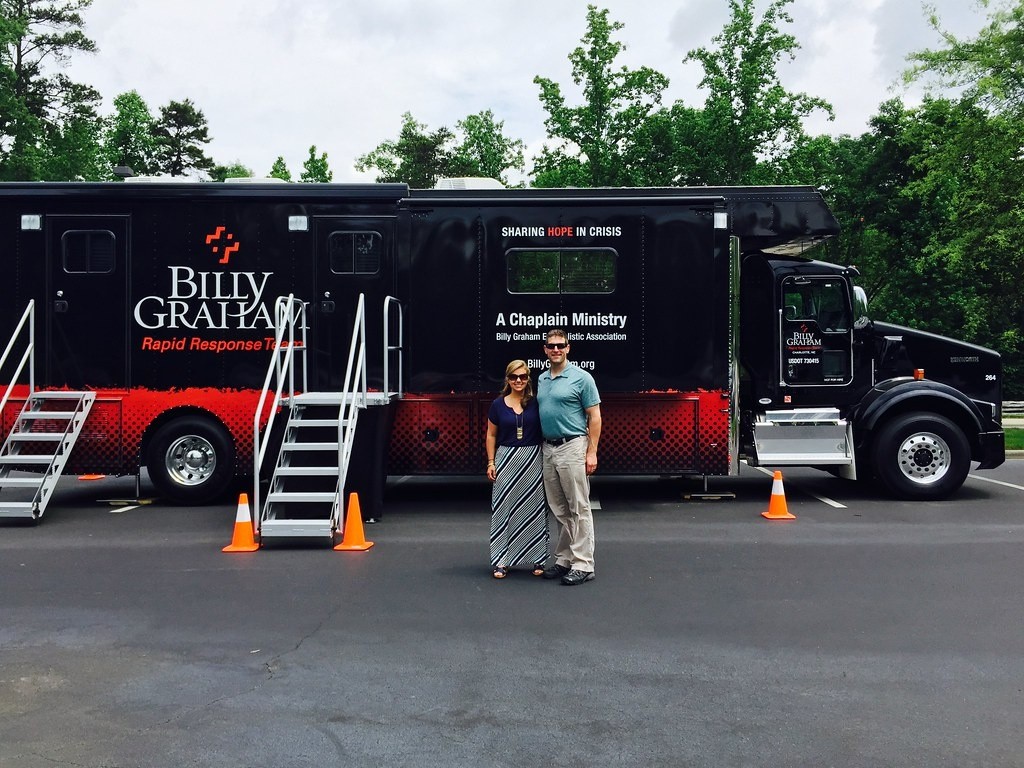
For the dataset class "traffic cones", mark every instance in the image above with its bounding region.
[761,469,796,520]
[77,474,106,480]
[221,493,263,552]
[332,491,374,552]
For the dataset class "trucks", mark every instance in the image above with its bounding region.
[0,178,1007,507]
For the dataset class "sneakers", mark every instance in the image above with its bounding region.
[560,568,596,586]
[541,564,570,580]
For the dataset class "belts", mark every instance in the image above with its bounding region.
[543,435,580,446]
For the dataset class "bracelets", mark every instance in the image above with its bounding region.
[487,457,495,467]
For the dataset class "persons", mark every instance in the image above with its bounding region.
[486,359,550,579]
[536,329,602,586]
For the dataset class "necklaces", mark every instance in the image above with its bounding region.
[514,413,525,441]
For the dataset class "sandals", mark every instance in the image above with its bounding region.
[532,563,545,577]
[493,565,508,579]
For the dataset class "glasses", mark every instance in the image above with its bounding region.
[545,343,567,350]
[507,373,528,381]
